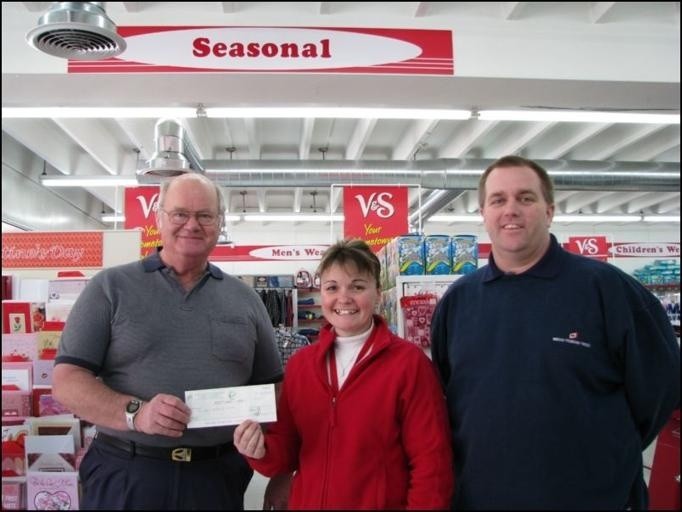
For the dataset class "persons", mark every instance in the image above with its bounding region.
[230,239,454,511]
[52,174,284,511]
[429,156,679,511]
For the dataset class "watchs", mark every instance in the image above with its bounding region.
[123,397,141,432]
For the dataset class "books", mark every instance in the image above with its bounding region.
[2,298,96,512]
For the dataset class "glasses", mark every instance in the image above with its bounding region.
[160,208,220,226]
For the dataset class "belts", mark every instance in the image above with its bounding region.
[95,432,235,462]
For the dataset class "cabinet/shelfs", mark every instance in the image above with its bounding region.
[297,288,328,340]
